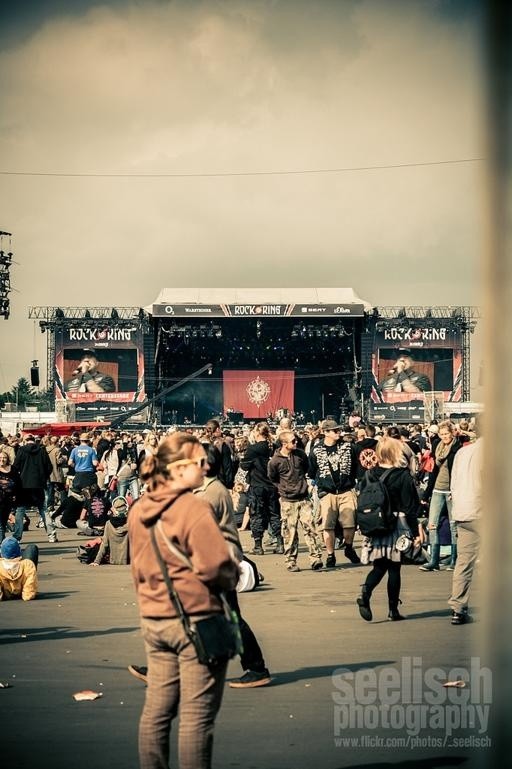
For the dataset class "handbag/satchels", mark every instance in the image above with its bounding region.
[191,616,236,663]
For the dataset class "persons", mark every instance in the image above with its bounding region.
[381,346,436,391]
[126,435,236,768]
[1,404,484,628]
[66,349,119,393]
[127,446,269,692]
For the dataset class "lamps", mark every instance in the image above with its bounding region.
[173,324,223,339]
[38,320,132,334]
[375,322,468,334]
[290,324,345,339]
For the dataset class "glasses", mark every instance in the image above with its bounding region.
[284,439,298,445]
[166,456,208,467]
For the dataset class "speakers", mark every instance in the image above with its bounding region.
[143,334,155,393]
[31,366,40,386]
[361,333,374,393]
[228,420,298,425]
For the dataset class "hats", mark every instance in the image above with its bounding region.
[1,537,21,559]
[322,421,340,430]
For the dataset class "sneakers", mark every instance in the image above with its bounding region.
[128,665,147,683]
[230,669,271,688]
[250,541,473,625]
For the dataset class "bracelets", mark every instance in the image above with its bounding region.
[397,371,409,382]
[82,372,93,382]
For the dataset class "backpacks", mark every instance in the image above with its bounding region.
[355,467,397,536]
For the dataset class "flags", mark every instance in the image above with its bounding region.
[222,370,295,420]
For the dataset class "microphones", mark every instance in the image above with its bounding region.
[387,366,398,377]
[72,366,82,376]
